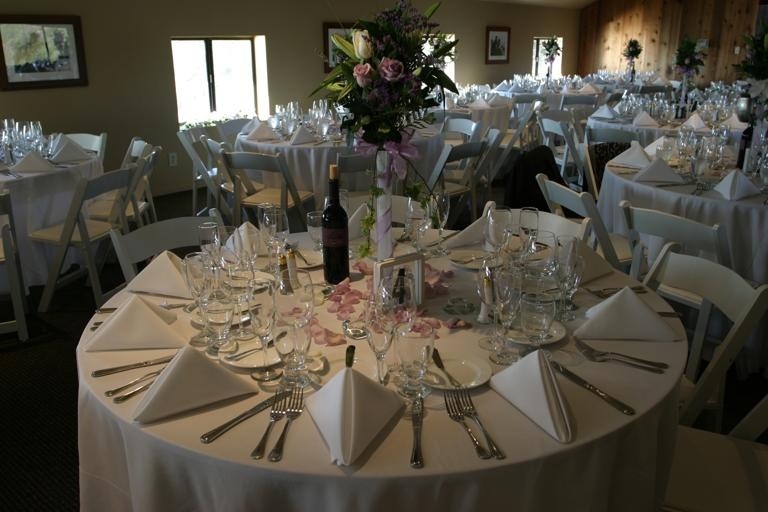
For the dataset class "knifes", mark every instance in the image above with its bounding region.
[548,360,636,418]
[93,300,187,315]
[410,395,429,469]
[200,391,293,446]
[90,350,182,379]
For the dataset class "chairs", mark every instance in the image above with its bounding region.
[0,72,768,512]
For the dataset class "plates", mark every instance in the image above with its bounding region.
[217,331,295,373]
[405,349,493,395]
[492,308,569,349]
[220,267,276,292]
[446,246,507,271]
[188,298,254,331]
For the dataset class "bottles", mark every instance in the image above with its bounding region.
[413,64,662,118]
[320,165,350,284]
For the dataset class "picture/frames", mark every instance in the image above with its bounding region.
[484,27,510,64]
[323,21,373,75]
[0,13,88,91]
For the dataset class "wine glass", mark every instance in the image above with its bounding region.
[245,91,348,146]
[0,116,89,180]
[600,81,768,201]
[182,187,585,410]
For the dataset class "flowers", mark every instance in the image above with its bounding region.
[621,38,643,60]
[539,33,564,62]
[672,35,711,76]
[731,33,768,80]
[304,0,459,258]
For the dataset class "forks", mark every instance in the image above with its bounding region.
[570,333,671,375]
[440,384,511,463]
[250,384,305,462]
[585,285,649,300]
